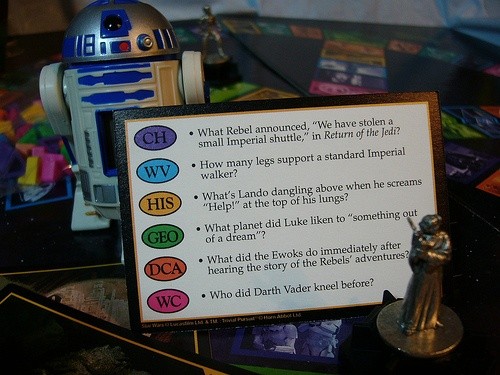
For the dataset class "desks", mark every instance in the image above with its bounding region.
[1,15,499,375]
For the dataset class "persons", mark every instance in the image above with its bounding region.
[396,210,451,335]
[196,3,230,63]
[253,321,300,356]
[298,319,343,358]
